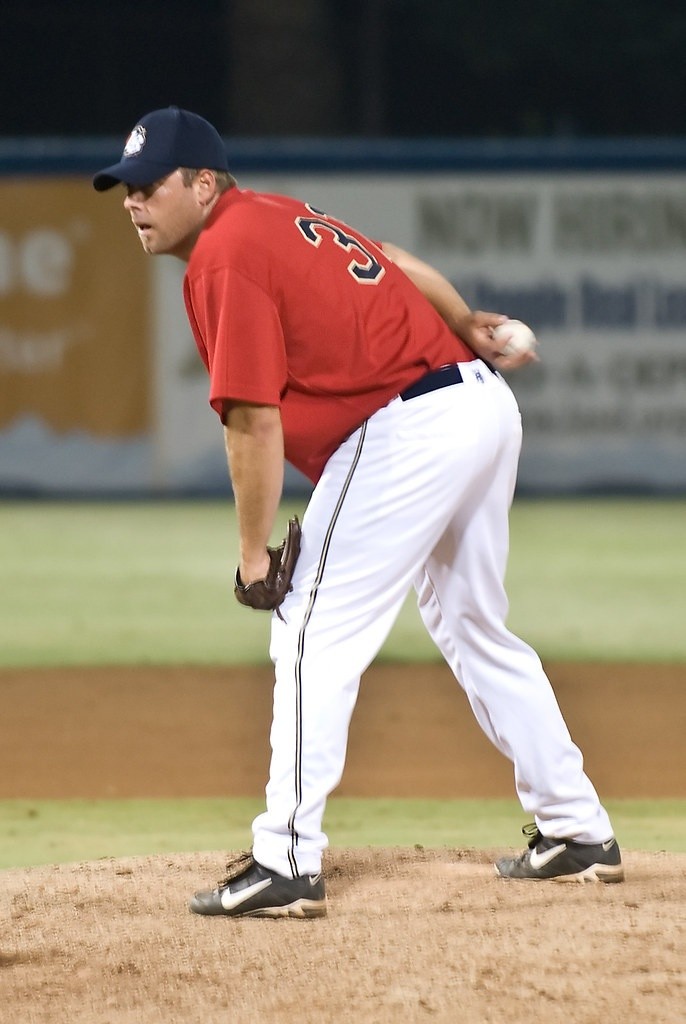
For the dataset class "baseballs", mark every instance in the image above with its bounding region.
[491,319,537,357]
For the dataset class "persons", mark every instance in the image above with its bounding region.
[92,107,625,916]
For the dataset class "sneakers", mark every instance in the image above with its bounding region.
[493,823,624,883]
[189,850,327,918]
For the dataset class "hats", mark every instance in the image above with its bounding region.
[93,105,228,191]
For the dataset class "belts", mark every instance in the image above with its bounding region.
[399,357,497,401]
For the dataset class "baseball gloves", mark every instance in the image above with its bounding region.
[234,514,304,613]
